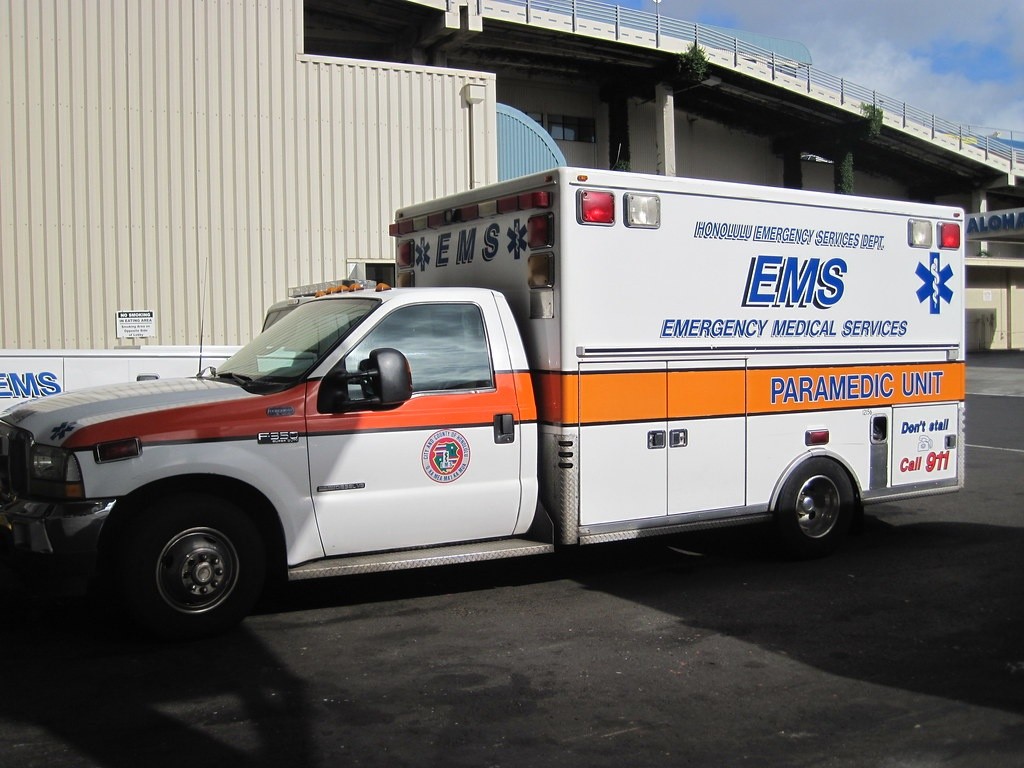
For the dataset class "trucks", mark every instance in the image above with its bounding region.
[0,167,966,637]
